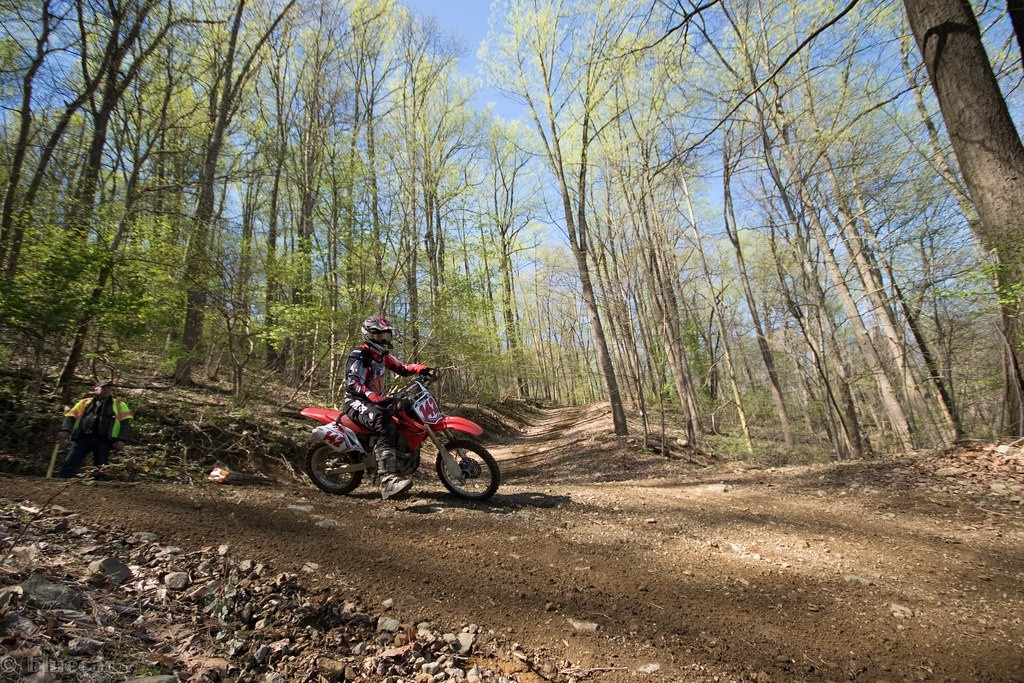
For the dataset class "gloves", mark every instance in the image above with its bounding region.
[364,390,389,404]
[408,363,429,375]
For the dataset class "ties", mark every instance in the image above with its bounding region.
[92,396,105,434]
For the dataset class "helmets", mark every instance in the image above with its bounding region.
[361,316,395,355]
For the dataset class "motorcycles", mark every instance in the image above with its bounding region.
[298,365,502,501]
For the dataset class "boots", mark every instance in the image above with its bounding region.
[374,447,413,501]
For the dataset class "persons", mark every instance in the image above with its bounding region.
[344,314,430,500]
[57,380,133,480]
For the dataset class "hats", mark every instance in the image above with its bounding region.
[96,380,114,387]
